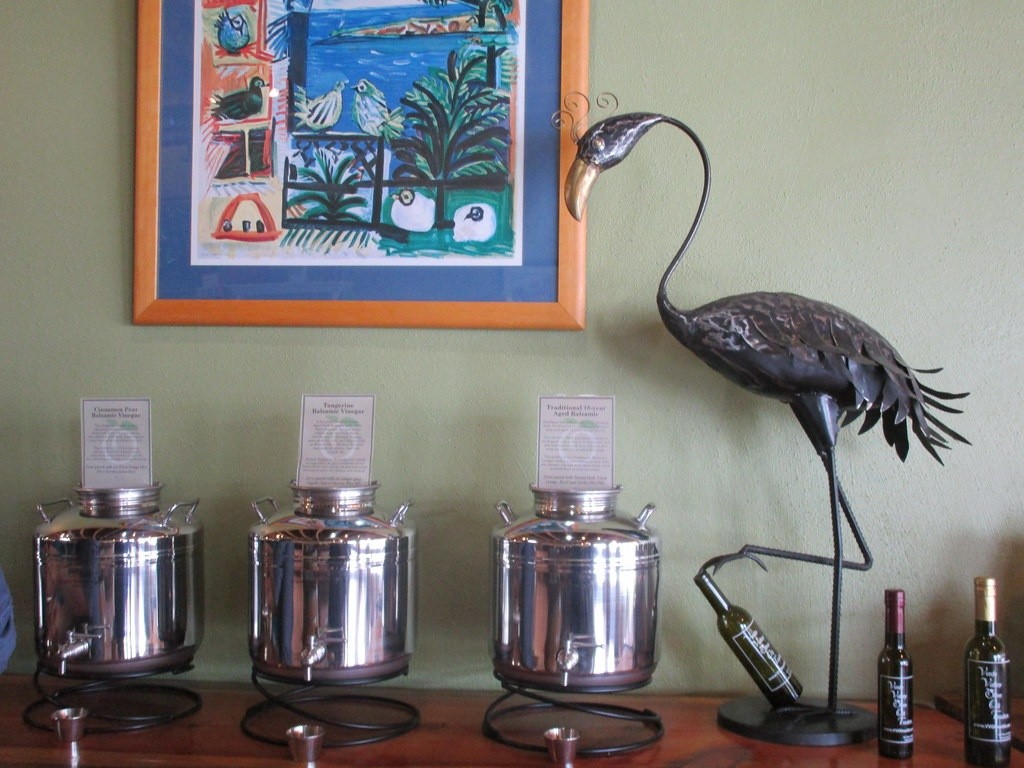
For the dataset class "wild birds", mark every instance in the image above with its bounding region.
[550,90,973,726]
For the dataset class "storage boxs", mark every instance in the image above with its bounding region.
[32,482,205,676]
[247,479,418,687]
[492,484,662,696]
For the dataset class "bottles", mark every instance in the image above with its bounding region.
[247,478,416,684]
[33,482,206,673]
[491,482,665,691]
[690,570,803,706]
[878,588,915,759]
[961,575,1015,768]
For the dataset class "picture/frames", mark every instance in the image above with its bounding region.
[131,0,591,331]
